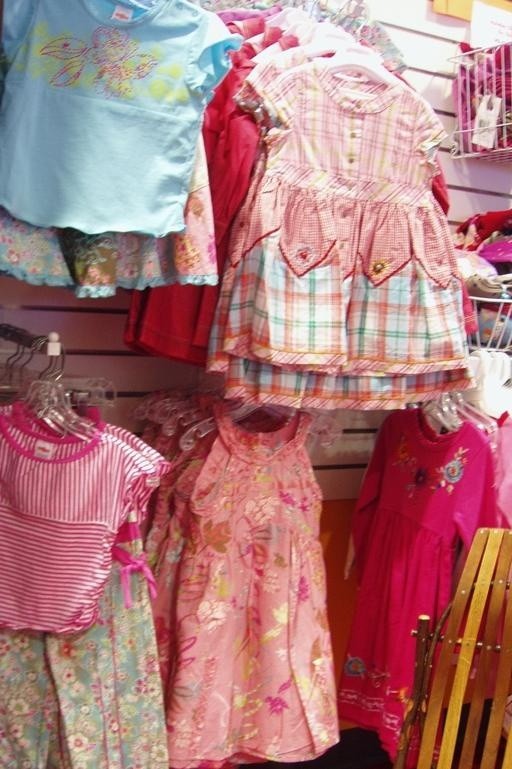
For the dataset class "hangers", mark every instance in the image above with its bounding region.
[223,0,433,107]
[376,373,500,453]
